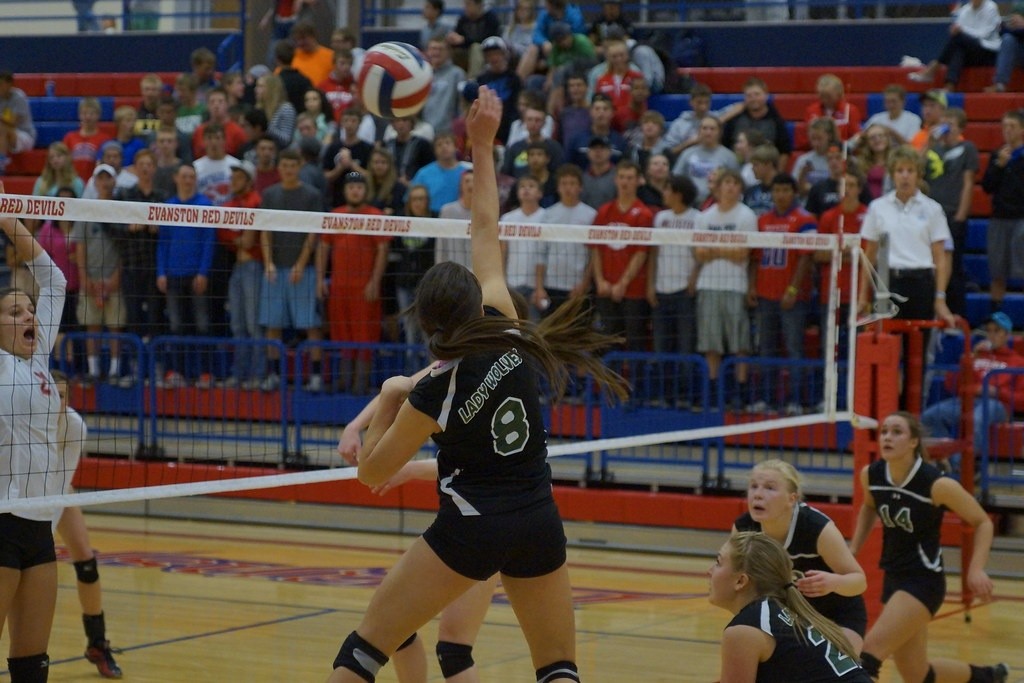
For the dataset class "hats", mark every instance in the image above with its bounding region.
[479,35,507,55]
[661,171,698,206]
[586,134,611,147]
[228,160,258,181]
[92,163,117,177]
[247,64,271,78]
[983,311,1012,333]
[917,87,948,110]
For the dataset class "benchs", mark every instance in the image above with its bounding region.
[10,67,1024,459]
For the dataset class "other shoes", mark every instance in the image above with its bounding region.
[79,369,372,397]
[552,382,819,418]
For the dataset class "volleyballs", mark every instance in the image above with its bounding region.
[355,40,434,119]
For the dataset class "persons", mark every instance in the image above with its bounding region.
[330,84,586,682]
[0,1,1024,495]
[704,532,870,683]
[0,219,68,683]
[728,459,868,660]
[847,411,1010,683]
[48,370,125,679]
[335,359,499,682]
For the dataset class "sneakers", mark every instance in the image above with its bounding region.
[84,639,122,679]
